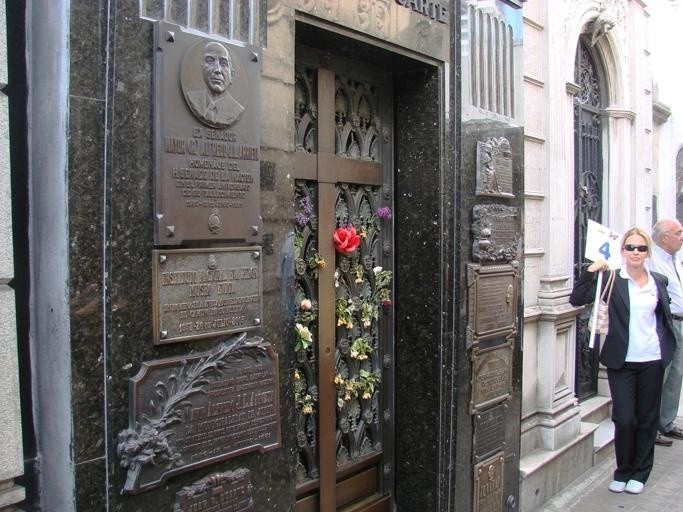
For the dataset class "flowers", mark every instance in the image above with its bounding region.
[291,195,393,413]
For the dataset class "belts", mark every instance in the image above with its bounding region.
[671,314,683,321]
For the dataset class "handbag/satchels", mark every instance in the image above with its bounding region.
[587,269,615,335]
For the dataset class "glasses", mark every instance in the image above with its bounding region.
[622,244,648,252]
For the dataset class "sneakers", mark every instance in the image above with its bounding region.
[608,480,644,493]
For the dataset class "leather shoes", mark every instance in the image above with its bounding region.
[655,431,673,446]
[663,427,683,440]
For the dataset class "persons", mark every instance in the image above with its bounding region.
[569,218,683,494]
